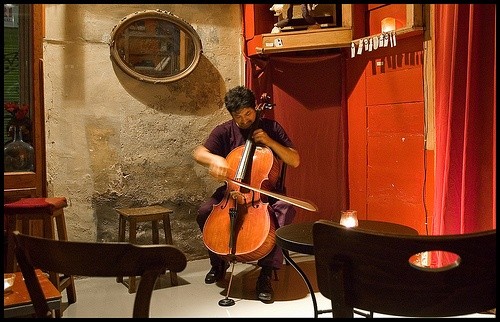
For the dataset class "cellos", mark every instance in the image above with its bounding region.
[201,91,282,306]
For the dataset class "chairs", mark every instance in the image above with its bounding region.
[313,218,497,319]
[277,159,290,266]
[12,228,188,318]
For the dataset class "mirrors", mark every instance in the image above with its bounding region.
[108,8,204,85]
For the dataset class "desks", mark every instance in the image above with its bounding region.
[4,268,63,318]
[275,219,420,318]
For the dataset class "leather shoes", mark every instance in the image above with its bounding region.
[256,274,273,301]
[204,263,229,284]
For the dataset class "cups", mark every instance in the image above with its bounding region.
[340,210,358,228]
[4,273,16,295]
[380,17,396,33]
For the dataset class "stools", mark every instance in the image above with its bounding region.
[116,206,179,295]
[4,197,77,317]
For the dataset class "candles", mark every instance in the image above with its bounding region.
[340,211,358,228]
[381,17,396,32]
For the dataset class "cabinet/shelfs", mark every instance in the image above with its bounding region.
[175,29,195,70]
[117,19,173,68]
[5,3,48,274]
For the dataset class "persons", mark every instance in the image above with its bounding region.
[192,85,301,303]
